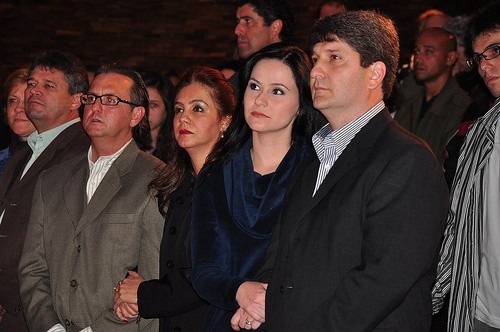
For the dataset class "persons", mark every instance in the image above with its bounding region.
[0,0,494,174]
[112,65,238,332]
[0,50,92,332]
[190,41,329,332]
[264,10,450,332]
[17,63,167,332]
[431,5,500,332]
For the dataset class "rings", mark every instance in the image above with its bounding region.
[245,319,253,325]
[113,281,122,291]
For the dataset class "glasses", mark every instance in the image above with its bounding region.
[80,94,138,106]
[466,46,500,71]
